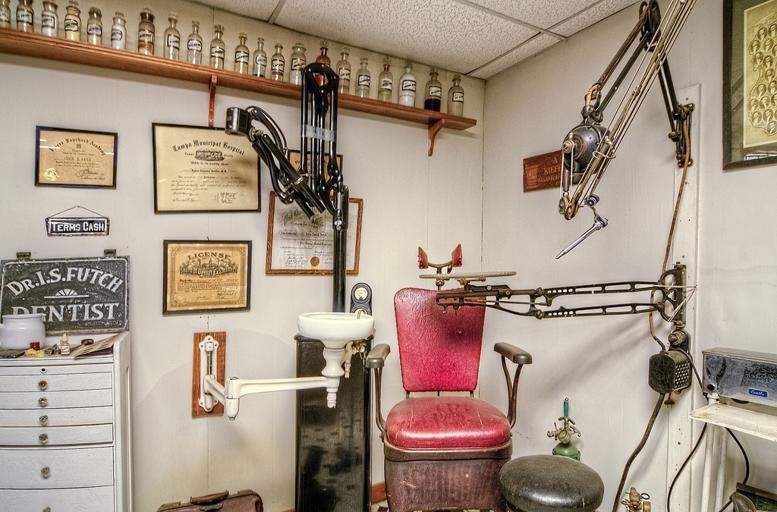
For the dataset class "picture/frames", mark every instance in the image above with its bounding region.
[287,149,343,196]
[163,240,252,315]
[723,0,777,170]
[266,191,363,275]
[152,122,261,213]
[35,126,118,188]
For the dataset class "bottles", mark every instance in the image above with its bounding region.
[315,46,330,88]
[86,6,103,45]
[269,44,285,81]
[137,7,156,56]
[187,20,202,66]
[424,67,441,114]
[14,0,37,33]
[356,59,371,98]
[378,57,392,103]
[447,74,464,117]
[1,0,12,29]
[335,48,350,93]
[111,10,126,51]
[62,0,81,42]
[59,331,71,356]
[208,23,225,70]
[398,63,417,107]
[288,43,306,84]
[41,0,61,37]
[163,11,180,61]
[233,32,249,75]
[251,37,266,78]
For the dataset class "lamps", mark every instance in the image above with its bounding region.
[555,0,695,260]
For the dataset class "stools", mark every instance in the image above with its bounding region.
[499,455,604,512]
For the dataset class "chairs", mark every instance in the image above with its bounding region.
[366,286,532,512]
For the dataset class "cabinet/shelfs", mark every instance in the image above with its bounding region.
[1,332,132,512]
